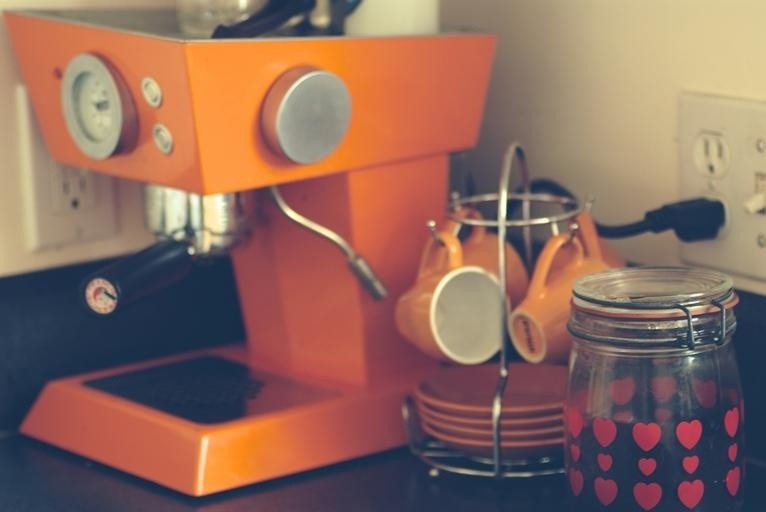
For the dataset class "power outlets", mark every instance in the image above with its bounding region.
[18,84,118,252]
[678,91,766,296]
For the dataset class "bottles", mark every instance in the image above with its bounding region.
[562,266,746,512]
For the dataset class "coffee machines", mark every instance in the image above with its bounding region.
[2,2,497,498]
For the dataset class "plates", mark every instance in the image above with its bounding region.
[412,364,573,454]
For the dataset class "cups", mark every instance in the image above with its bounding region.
[395,231,511,365]
[508,235,611,364]
[575,209,627,269]
[441,209,529,311]
